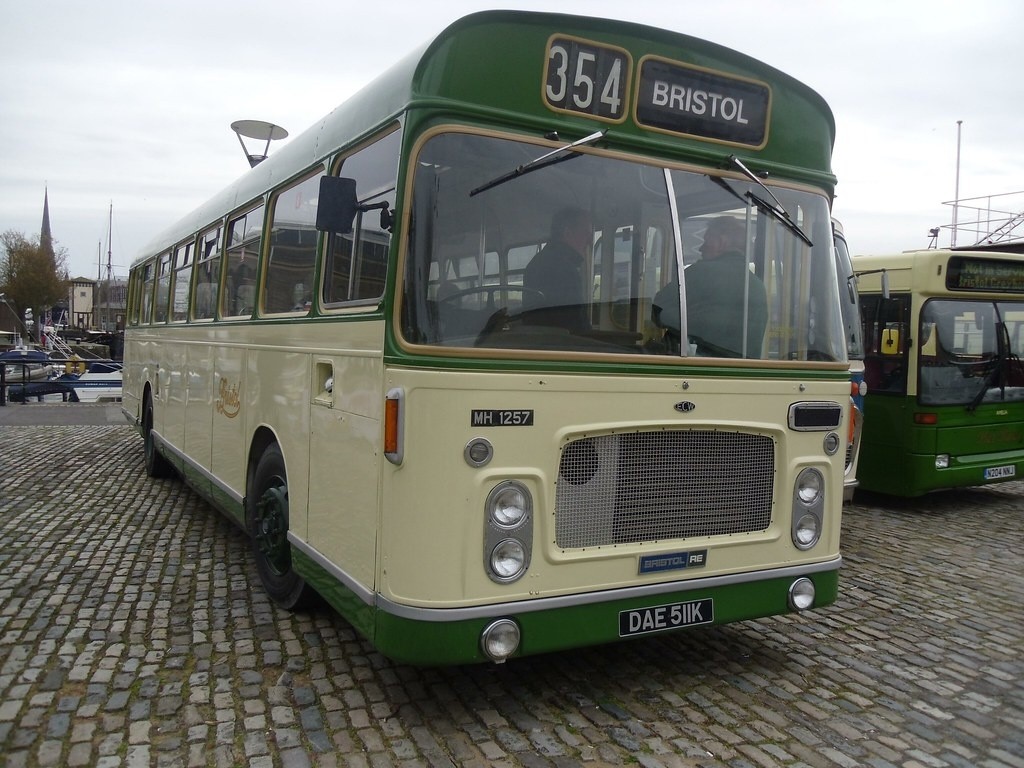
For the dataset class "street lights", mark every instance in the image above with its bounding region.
[954,121,963,248]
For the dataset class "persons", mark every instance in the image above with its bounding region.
[653,218,770,357]
[523,210,602,335]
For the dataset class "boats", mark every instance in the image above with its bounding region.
[0,292,123,402]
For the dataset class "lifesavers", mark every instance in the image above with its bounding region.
[65,356,85,374]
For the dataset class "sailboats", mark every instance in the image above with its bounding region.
[54,198,125,360]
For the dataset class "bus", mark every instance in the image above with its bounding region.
[427,191,1024,496]
[120,10,851,664]
